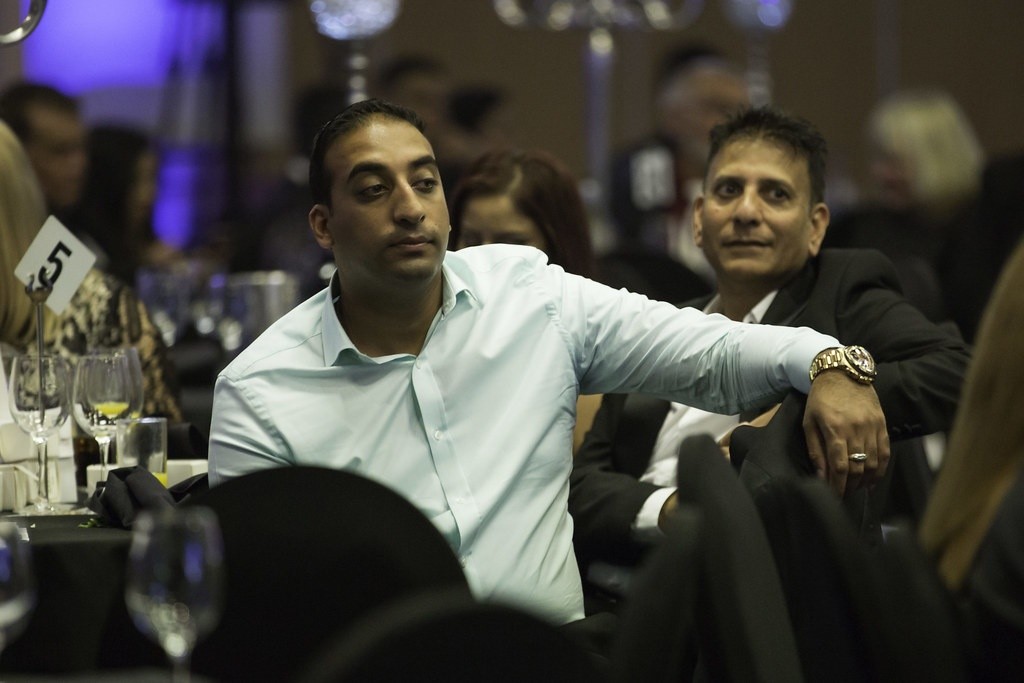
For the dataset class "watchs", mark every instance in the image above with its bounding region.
[809,345,878,386]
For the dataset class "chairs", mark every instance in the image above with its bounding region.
[93,424,969,683]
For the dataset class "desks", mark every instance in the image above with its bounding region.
[0,514,140,683]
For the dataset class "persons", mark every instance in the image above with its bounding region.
[568,105,971,564]
[209,41,891,627]
[0,82,202,457]
[840,87,1024,344]
[447,147,604,460]
[917,237,1024,683]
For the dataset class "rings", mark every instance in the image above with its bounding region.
[848,453,868,463]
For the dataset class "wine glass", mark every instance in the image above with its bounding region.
[71,358,143,515]
[127,515,219,683]
[86,346,142,465]
[9,354,70,516]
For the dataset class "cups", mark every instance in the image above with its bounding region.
[116,417,167,487]
